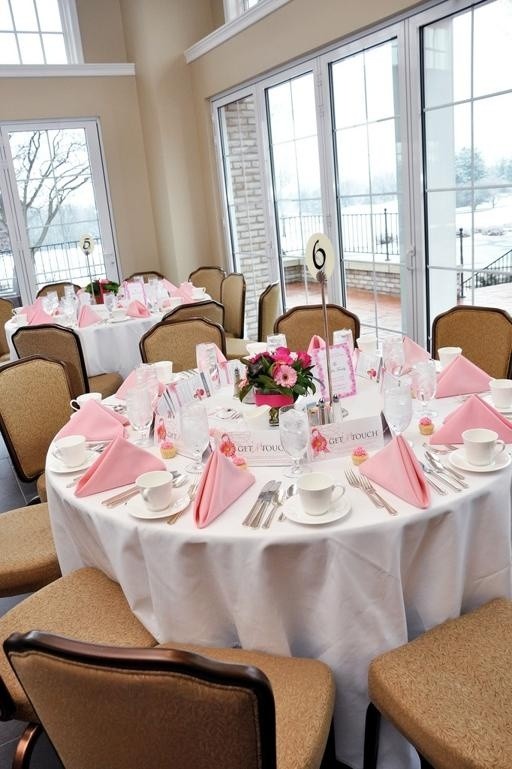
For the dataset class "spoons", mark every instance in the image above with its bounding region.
[428,460,470,489]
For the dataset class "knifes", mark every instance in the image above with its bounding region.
[241,479,275,528]
[249,481,284,529]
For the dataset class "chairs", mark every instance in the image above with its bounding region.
[364,597,512,769]
[4,631,337,769]
[161,300,224,329]
[36,282,82,301]
[188,265,227,303]
[123,272,165,283]
[0,354,76,502]
[220,272,246,338]
[139,316,228,373]
[0,503,61,597]
[11,323,123,411]
[274,304,360,353]
[432,304,512,379]
[0,567,158,769]
[0,298,15,363]
[226,279,280,360]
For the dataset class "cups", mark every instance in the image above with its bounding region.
[331,329,354,354]
[148,277,167,316]
[411,357,437,421]
[11,307,24,316]
[278,404,310,478]
[437,346,463,372]
[460,428,506,467]
[63,284,76,299]
[212,362,236,418]
[355,335,378,353]
[170,297,186,307]
[112,307,126,318]
[52,435,89,468]
[42,295,54,315]
[16,313,28,325]
[135,470,173,513]
[192,287,207,297]
[295,473,346,516]
[195,342,217,378]
[151,361,174,385]
[177,402,210,475]
[60,295,77,328]
[245,342,269,357]
[489,379,511,408]
[383,380,413,450]
[71,392,102,411]
[47,290,60,309]
[103,291,117,314]
[383,338,408,379]
[267,334,288,351]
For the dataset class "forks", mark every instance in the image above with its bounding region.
[357,471,399,518]
[168,472,204,525]
[342,464,384,510]
[422,442,456,454]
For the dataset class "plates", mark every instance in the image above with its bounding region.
[127,487,190,521]
[193,297,206,302]
[449,445,512,474]
[107,316,130,324]
[280,493,352,526]
[49,450,100,474]
